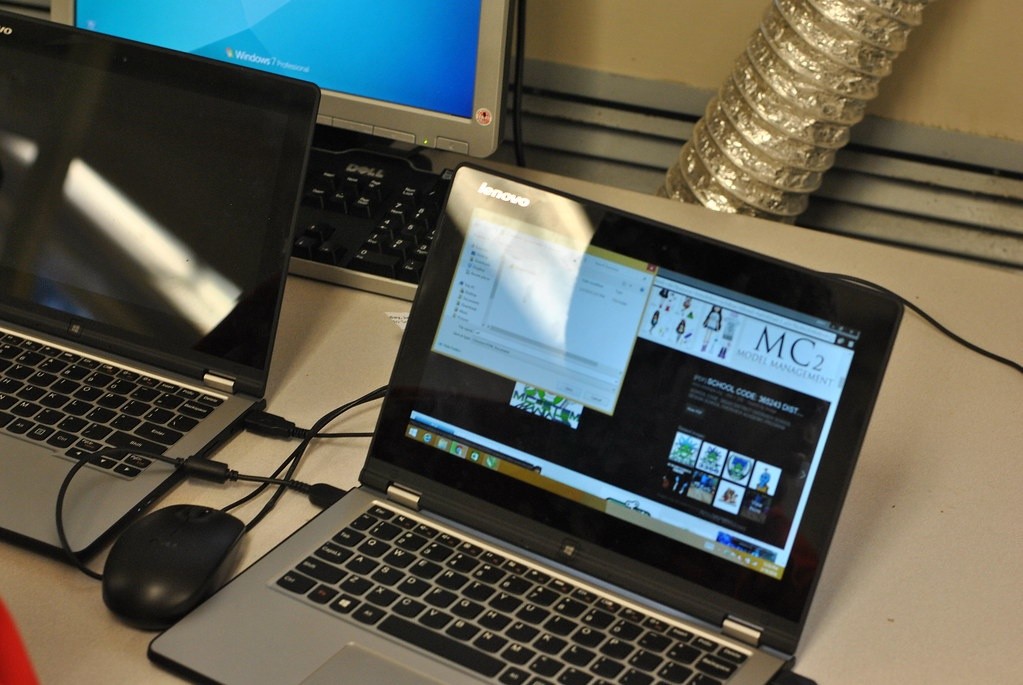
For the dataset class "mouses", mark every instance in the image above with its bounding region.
[102,503,247,632]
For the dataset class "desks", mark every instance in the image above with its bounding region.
[2,157,1023,685]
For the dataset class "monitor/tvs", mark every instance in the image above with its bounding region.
[48,0,515,160]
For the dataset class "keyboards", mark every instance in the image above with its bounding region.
[288,141,456,302]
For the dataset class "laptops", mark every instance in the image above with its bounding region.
[146,162,906,685]
[0,8,322,570]
